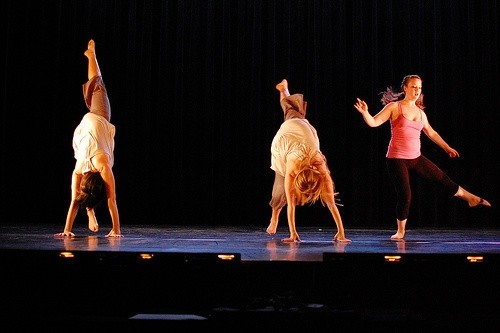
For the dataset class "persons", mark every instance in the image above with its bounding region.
[265,77,352,245]
[54,38,123,241]
[351,73,495,241]
